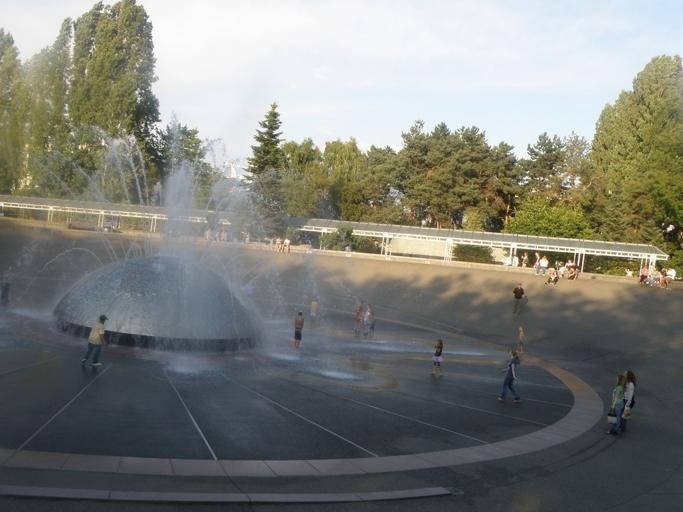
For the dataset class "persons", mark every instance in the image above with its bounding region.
[495,350,521,403]
[364,304,374,337]
[294,311,303,348]
[274,237,282,254]
[429,339,443,375]
[603,375,625,435]
[617,369,635,431]
[310,297,317,318]
[280,236,290,253]
[520,251,580,287]
[81,315,109,367]
[352,299,363,335]
[624,264,676,289]
[511,283,524,314]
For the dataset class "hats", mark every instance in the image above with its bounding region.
[100,315,108,320]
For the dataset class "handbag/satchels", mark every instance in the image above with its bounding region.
[622,405,632,419]
[432,356,444,362]
[624,399,635,407]
[608,413,617,423]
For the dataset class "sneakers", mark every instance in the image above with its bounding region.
[498,396,523,403]
[606,426,624,434]
[81,359,103,366]
